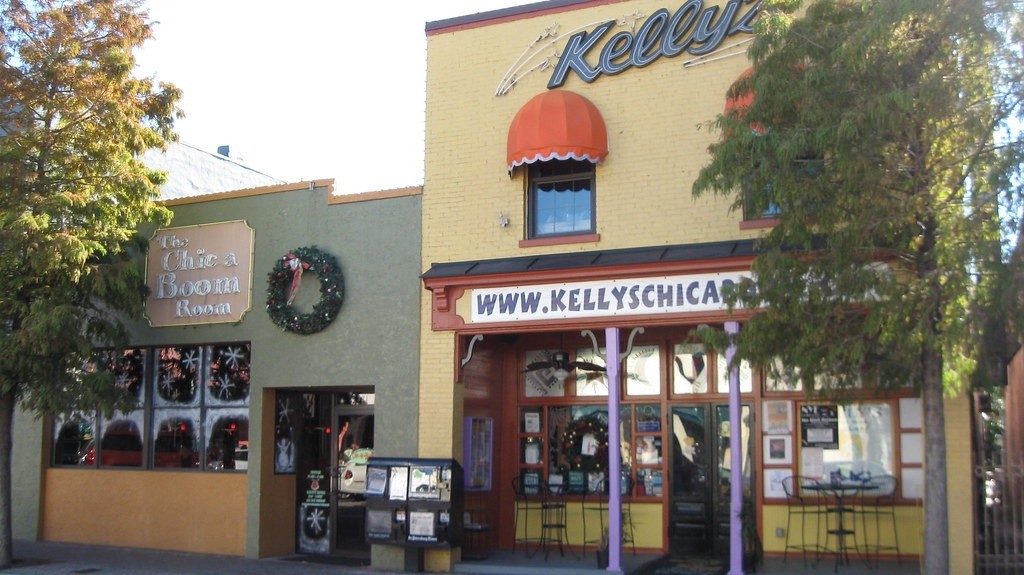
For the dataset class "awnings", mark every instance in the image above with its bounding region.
[506,90,609,179]
[723,62,863,145]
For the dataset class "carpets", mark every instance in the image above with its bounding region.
[633,553,730,575]
[280,553,371,567]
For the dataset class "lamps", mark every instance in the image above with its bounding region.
[548,367,571,382]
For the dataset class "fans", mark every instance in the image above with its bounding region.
[520,333,606,373]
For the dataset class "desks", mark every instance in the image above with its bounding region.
[800,483,878,573]
[526,484,581,562]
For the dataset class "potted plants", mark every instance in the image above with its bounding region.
[596,511,636,569]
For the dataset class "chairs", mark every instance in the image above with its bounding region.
[845,475,898,572]
[581,475,635,555]
[512,475,563,556]
[781,475,843,566]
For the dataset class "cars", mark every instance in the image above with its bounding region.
[330,461,368,501]
